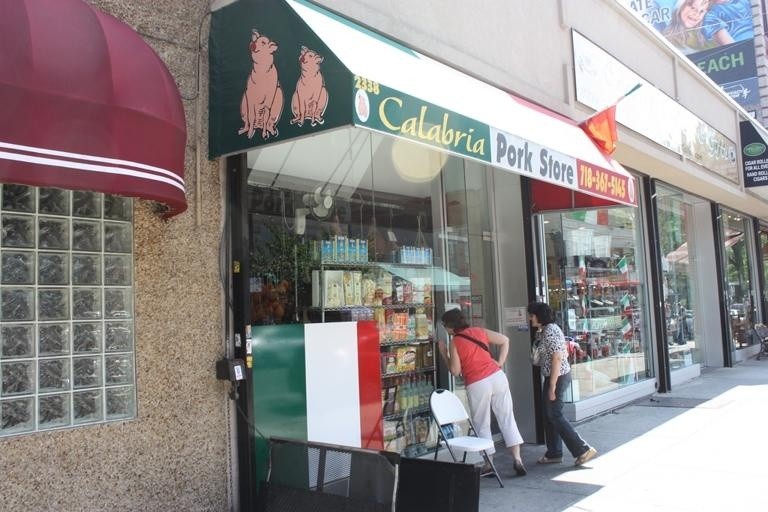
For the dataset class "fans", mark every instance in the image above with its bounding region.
[291,182,336,235]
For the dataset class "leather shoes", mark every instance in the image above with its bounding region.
[512,460,527,475]
[480,467,496,478]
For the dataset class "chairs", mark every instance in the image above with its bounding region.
[429,387,505,489]
[752,322,768,360]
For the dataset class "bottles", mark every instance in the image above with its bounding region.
[400,244,434,266]
[395,416,455,449]
[384,373,435,412]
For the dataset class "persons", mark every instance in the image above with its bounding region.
[438,308,527,478]
[528,302,597,465]
[663,0,752,54]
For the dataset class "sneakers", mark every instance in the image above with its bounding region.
[574,446,597,466]
[537,455,564,463]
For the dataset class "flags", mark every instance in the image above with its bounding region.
[579,104,619,155]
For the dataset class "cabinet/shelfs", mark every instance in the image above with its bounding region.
[293,243,435,456]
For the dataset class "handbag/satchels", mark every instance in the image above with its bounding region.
[530,346,541,367]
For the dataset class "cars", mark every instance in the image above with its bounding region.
[729,303,744,316]
